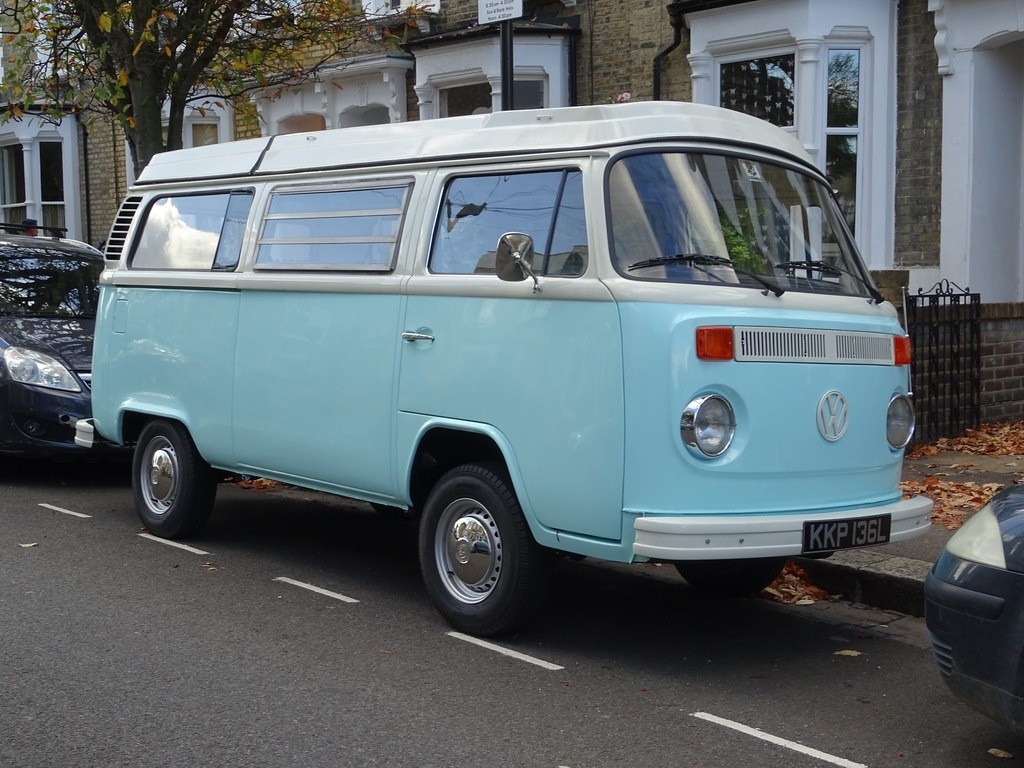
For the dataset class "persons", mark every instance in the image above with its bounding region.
[19,219,39,236]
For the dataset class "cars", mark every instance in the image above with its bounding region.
[0,220,135,469]
[920,481,1024,752]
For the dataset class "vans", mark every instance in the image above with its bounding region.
[70,99,935,642]
